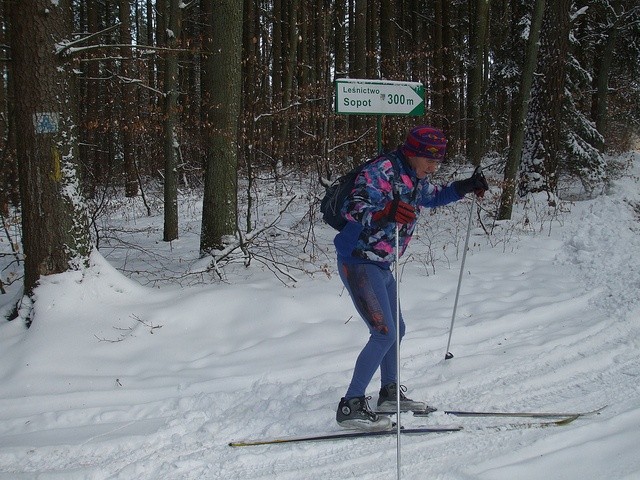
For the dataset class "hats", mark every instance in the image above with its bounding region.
[403,128,447,160]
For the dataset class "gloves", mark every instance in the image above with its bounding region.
[373,199,416,227]
[454,173,487,198]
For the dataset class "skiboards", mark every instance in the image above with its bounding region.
[229,404,610,446]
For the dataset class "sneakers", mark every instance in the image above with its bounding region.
[335,395,392,432]
[377,383,430,412]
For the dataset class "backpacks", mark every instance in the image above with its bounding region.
[320,154,402,232]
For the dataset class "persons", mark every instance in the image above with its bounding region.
[333,127,488,433]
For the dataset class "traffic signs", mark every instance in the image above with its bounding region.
[336,79,425,117]
